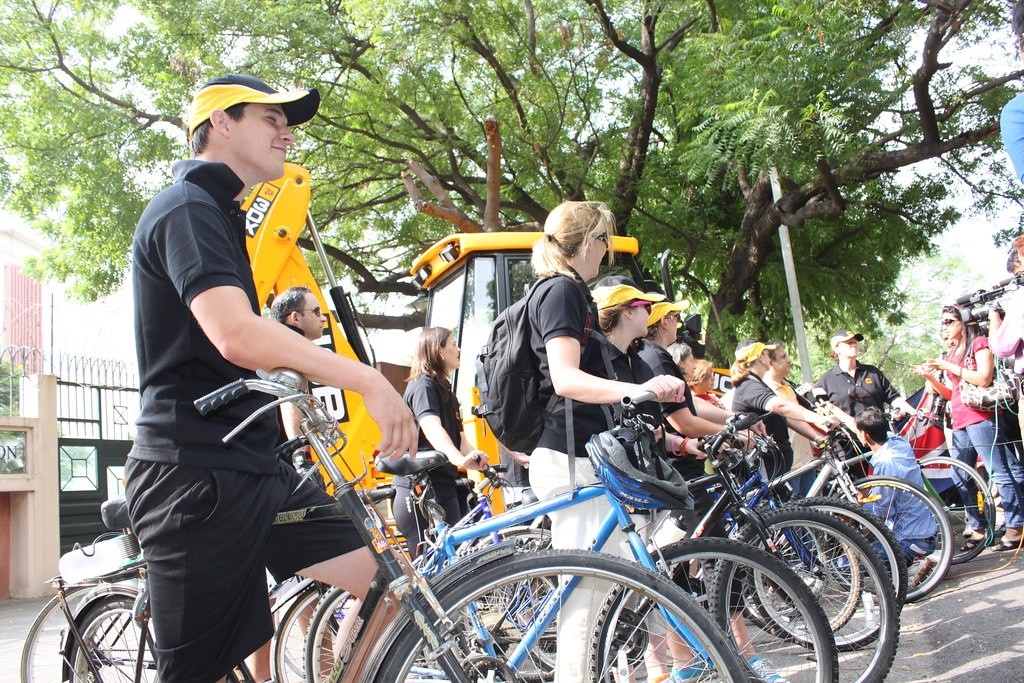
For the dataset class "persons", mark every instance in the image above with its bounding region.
[815,329,918,479]
[999,0,1024,184]
[527,199,685,683]
[125,77,418,683]
[908,305,1024,551]
[817,400,939,588]
[246,287,335,683]
[392,327,489,564]
[588,275,840,683]
[986,233,1024,446]
[498,442,552,551]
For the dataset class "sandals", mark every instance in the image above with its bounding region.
[960,535,995,551]
[992,538,1024,550]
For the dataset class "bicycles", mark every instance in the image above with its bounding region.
[21,372,999,683]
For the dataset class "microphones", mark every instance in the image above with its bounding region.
[795,382,830,415]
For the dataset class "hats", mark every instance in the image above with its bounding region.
[831,329,864,348]
[593,284,667,310]
[187,74,319,140]
[646,300,690,327]
[735,342,777,365]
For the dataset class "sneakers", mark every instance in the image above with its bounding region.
[748,659,791,683]
[671,660,716,683]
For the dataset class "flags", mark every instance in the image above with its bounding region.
[865,391,984,494]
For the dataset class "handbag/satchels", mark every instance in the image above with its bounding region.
[959,337,1014,411]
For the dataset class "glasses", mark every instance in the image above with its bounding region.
[626,299,651,314]
[591,231,609,247]
[285,307,320,319]
[941,319,958,326]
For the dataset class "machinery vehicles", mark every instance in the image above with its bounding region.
[231,160,736,545]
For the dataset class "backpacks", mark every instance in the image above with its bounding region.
[477,276,615,453]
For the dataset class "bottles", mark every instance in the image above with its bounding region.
[645,515,688,553]
[58,535,143,586]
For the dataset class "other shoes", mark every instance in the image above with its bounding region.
[906,557,933,591]
[995,524,1006,537]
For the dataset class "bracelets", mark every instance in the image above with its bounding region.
[680,437,690,456]
[671,436,682,456]
[960,367,962,377]
